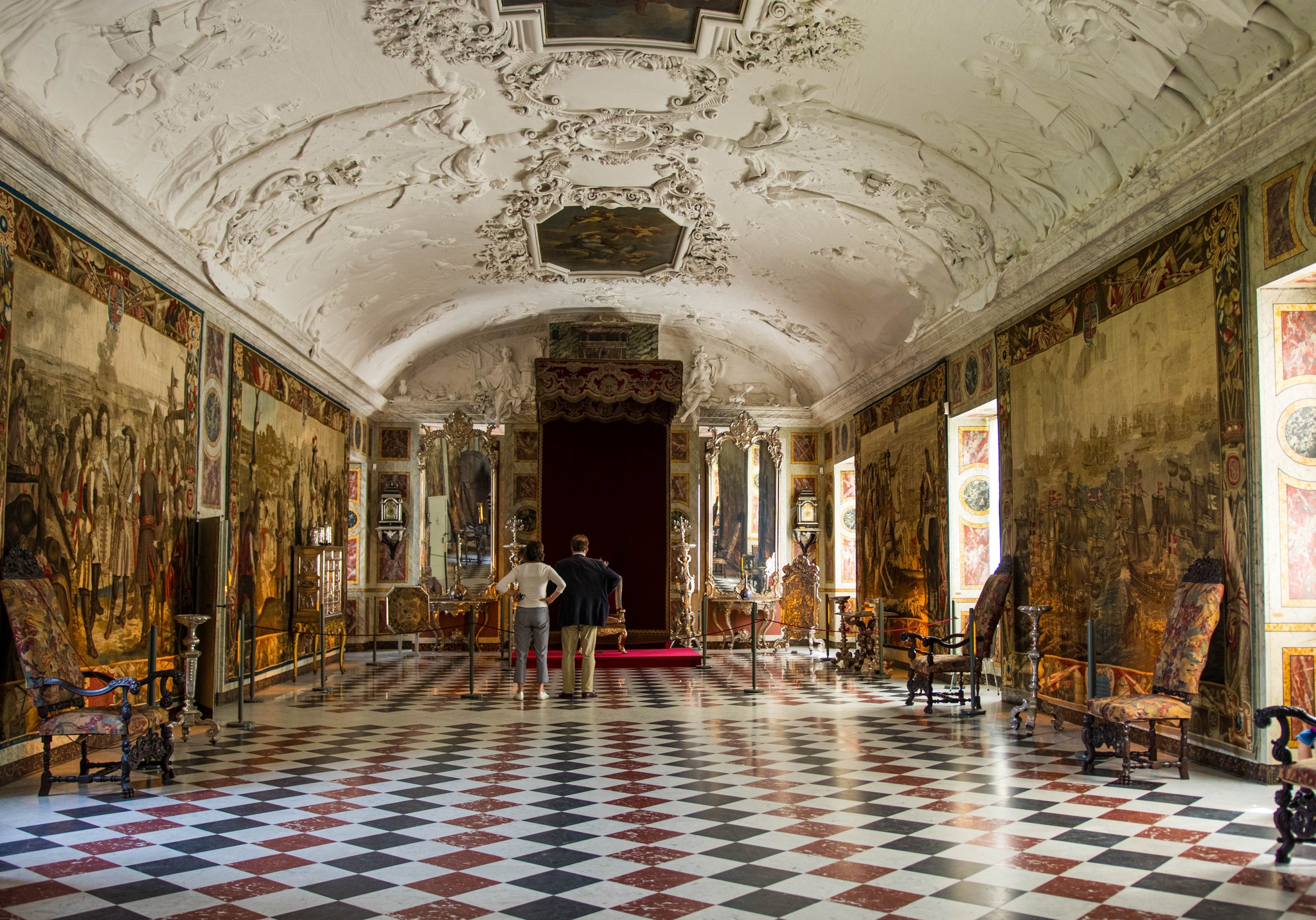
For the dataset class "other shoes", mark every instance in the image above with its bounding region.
[537,690,550,699]
[513,691,524,700]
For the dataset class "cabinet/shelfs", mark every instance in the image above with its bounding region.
[291,545,348,681]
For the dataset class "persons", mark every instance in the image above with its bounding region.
[480,344,531,427]
[495,540,566,701]
[679,352,725,432]
[550,534,620,700]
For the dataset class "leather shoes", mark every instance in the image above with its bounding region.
[582,690,598,699]
[559,692,573,698]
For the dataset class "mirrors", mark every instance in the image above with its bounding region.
[703,410,784,601]
[417,409,499,601]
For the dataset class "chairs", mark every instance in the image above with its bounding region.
[1082,556,1224,784]
[902,553,1014,715]
[712,512,744,578]
[576,558,627,654]
[950,625,999,695]
[1254,705,1316,866]
[0,546,186,797]
[447,503,485,567]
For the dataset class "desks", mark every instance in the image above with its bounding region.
[747,569,760,591]
[711,598,776,650]
[835,611,898,676]
[431,601,490,652]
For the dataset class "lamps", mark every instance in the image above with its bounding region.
[669,516,702,650]
[502,516,526,652]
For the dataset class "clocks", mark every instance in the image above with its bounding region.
[374,487,407,559]
[792,486,822,556]
